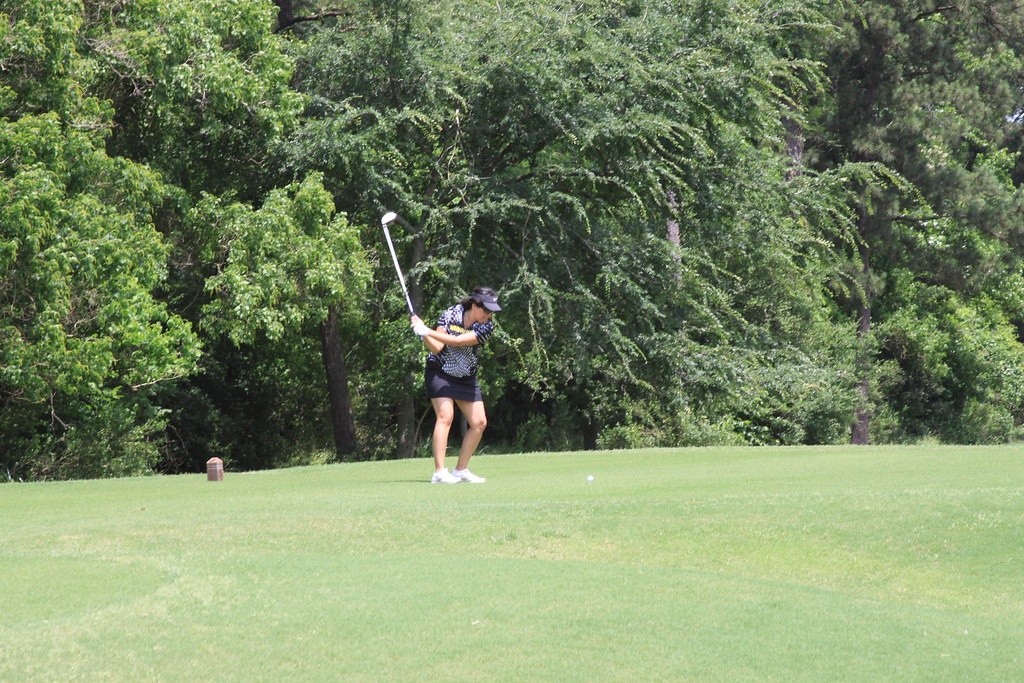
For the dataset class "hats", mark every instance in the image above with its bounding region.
[472,288,502,312]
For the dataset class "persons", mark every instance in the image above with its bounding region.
[410,287,501,484]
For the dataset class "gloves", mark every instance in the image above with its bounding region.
[410,314,430,341]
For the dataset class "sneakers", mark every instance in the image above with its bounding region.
[431,467,461,484]
[451,468,486,484]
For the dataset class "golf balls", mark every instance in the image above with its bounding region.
[586,476,594,481]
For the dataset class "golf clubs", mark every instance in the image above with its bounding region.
[382,211,425,343]
